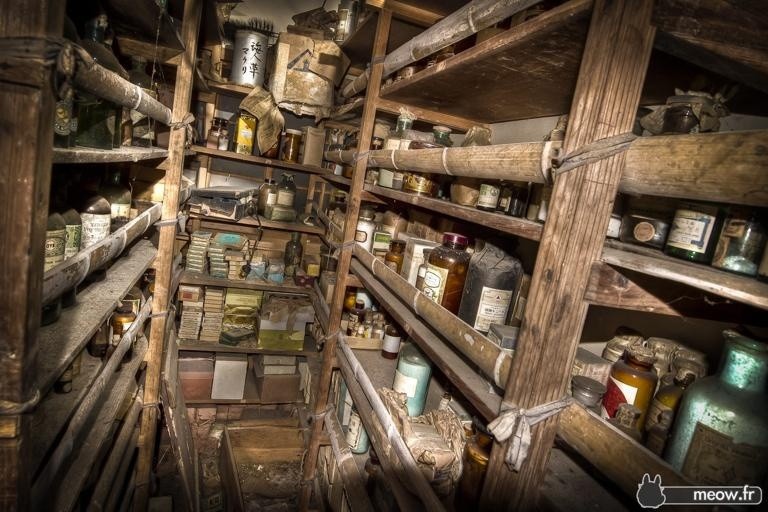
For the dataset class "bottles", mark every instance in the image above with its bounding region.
[42,6,158,394]
[206,116,303,279]
[321,107,767,512]
